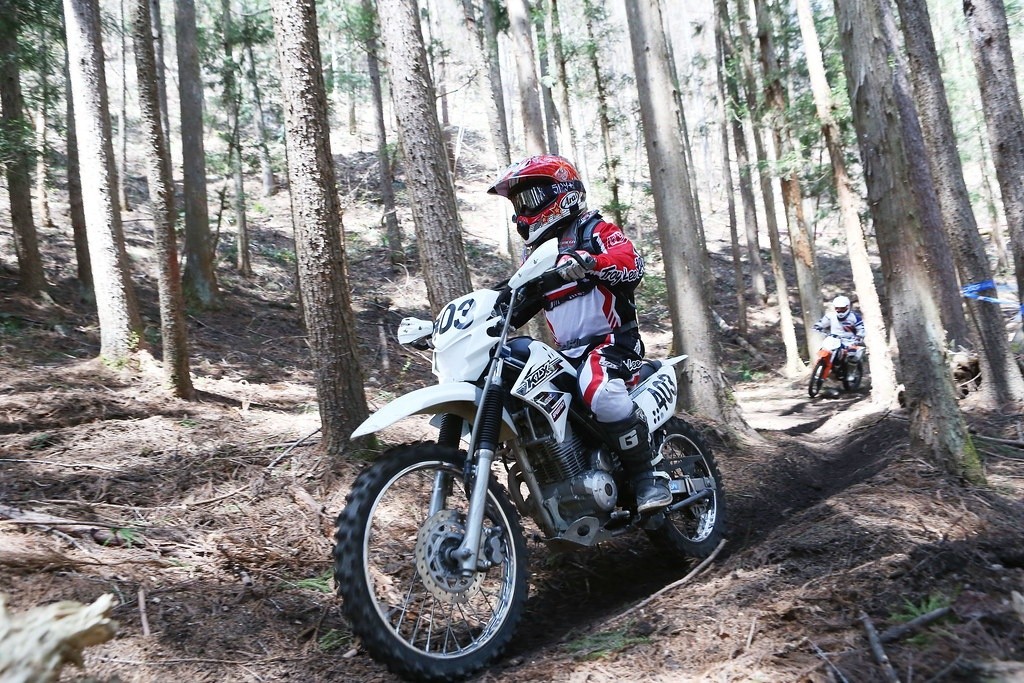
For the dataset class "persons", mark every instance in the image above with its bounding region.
[813,295,865,382]
[410,154,673,515]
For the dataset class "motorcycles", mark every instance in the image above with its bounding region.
[808,322,866,399]
[330,237,724,683]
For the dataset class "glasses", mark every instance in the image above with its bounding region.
[511,180,586,218]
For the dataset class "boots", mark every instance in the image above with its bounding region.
[597,400,673,513]
[846,362,858,382]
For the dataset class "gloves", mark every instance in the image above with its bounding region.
[557,250,591,282]
[813,324,823,331]
[854,337,862,344]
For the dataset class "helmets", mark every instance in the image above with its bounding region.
[832,296,851,320]
[487,154,586,246]
